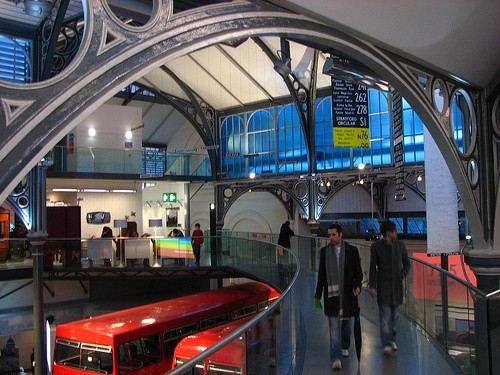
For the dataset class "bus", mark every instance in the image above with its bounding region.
[50,281,281,375]
[171,302,279,375]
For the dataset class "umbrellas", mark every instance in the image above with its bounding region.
[353,286,361,362]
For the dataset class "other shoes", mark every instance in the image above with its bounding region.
[332,358,341,369]
[383,345,392,354]
[392,343,398,350]
[342,349,349,357]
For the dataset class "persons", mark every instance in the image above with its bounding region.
[278,220,294,263]
[46,315,55,327]
[313,223,363,371]
[101,226,112,266]
[168,229,184,264]
[191,223,204,266]
[369,220,410,354]
[8,222,33,260]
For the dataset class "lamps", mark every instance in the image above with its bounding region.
[275,50,294,77]
[52,188,137,192]
